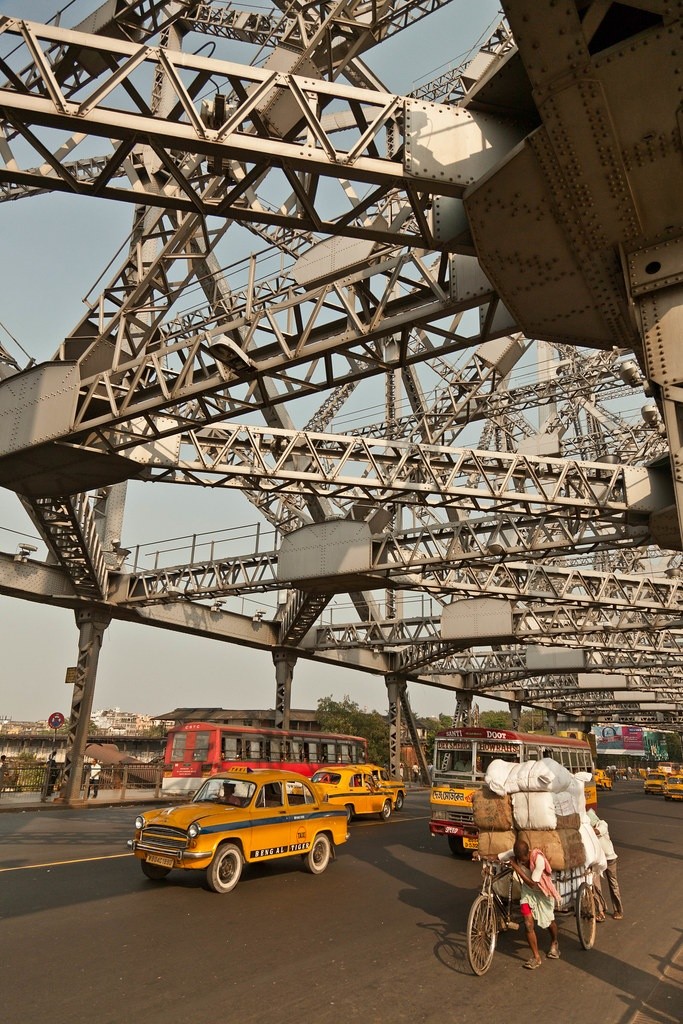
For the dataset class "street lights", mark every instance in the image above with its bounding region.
[364,705,368,714]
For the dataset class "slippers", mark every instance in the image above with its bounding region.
[523,958,542,969]
[548,950,561,959]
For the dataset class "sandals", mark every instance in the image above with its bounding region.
[595,914,606,921]
[613,912,624,919]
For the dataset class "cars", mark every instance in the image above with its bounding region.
[348,764,406,811]
[663,775,683,801]
[126,766,352,894]
[637,768,645,775]
[647,768,663,774]
[594,769,614,791]
[291,767,394,825]
[679,768,683,775]
[643,772,668,795]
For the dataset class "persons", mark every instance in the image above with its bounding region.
[356,776,379,794]
[216,780,241,806]
[221,752,225,760]
[587,809,623,921]
[85,759,102,800]
[453,752,472,772]
[509,838,563,970]
[408,760,435,782]
[605,763,654,781]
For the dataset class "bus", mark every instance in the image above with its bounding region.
[658,762,680,777]
[429,727,598,858]
[160,722,369,807]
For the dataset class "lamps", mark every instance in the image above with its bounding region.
[207,335,258,373]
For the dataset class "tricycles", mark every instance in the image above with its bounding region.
[466,851,597,977]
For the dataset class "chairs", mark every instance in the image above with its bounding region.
[452,760,472,779]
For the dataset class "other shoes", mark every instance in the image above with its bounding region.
[91,796,97,799]
